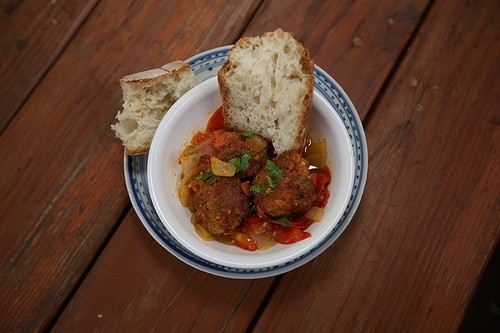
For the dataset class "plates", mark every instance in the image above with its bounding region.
[122,43,369,279]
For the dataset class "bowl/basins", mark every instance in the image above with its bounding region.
[146,73,356,269]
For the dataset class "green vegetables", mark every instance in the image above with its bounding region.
[194,129,291,228]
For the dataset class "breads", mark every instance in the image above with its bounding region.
[218,29,314,155]
[110,59,197,155]
